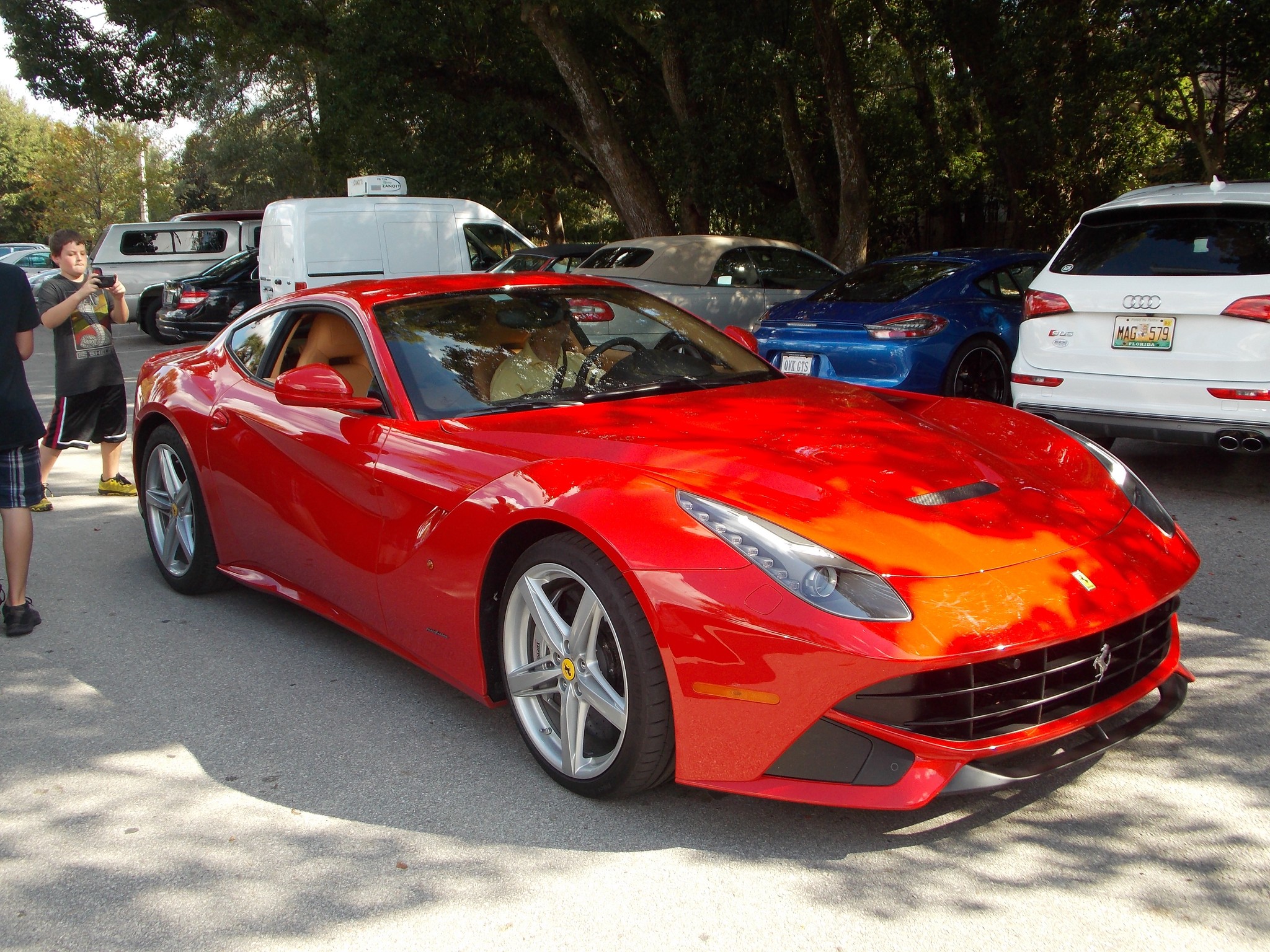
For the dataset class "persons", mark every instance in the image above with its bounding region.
[490,310,614,407]
[30,226,139,512]
[1,261,45,637]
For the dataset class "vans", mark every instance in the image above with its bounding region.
[170,209,268,223]
[257,197,573,313]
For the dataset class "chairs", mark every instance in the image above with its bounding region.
[297,314,376,399]
[469,303,585,414]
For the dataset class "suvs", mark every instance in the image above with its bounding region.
[1012,174,1270,461]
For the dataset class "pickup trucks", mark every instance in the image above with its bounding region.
[89,219,265,344]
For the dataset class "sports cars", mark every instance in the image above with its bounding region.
[132,289,1203,809]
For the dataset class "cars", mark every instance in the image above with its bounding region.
[0,242,90,323]
[157,246,260,339]
[487,234,1053,408]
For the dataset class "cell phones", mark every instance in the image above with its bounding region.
[92,275,116,288]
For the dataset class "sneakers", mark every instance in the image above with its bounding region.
[97,472,138,496]
[2,596,42,636]
[0,579,5,605]
[29,482,54,512]
